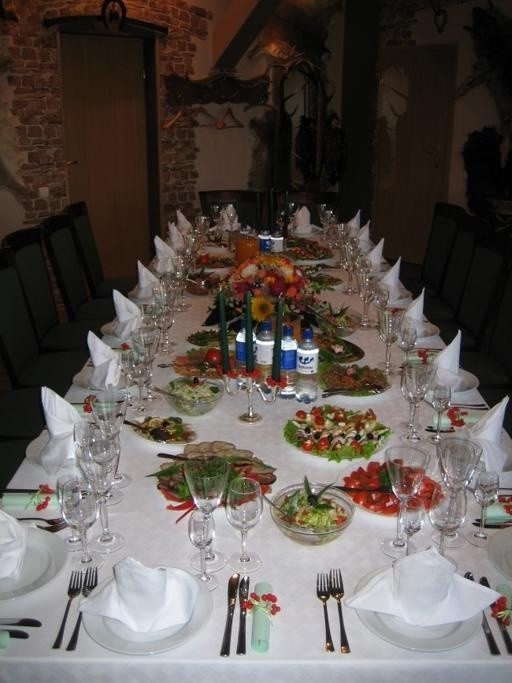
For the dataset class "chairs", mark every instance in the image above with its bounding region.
[1,357,65,435]
[198,189,266,232]
[2,249,91,385]
[38,216,120,321]
[273,188,338,229]
[3,224,110,351]
[2,441,36,495]
[61,203,142,298]
[390,198,512,442]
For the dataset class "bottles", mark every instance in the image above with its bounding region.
[236,316,320,404]
[235,219,283,258]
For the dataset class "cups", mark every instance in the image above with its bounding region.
[72,421,98,449]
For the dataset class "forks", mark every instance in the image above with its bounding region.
[53,564,100,653]
[16,513,68,532]
[316,572,337,653]
[421,398,489,409]
[328,568,351,653]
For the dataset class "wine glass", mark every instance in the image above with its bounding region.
[210,199,237,240]
[75,441,126,555]
[56,473,84,542]
[179,456,264,583]
[91,427,118,500]
[129,352,154,413]
[276,199,299,244]
[316,200,452,443]
[118,347,138,406]
[63,480,106,572]
[90,391,129,482]
[379,435,499,559]
[131,213,209,402]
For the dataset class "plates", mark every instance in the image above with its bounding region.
[72,367,140,393]
[134,419,198,446]
[336,458,444,518]
[100,318,159,340]
[488,526,511,581]
[82,564,214,655]
[453,366,479,396]
[293,223,322,238]
[1,524,65,598]
[355,565,484,651]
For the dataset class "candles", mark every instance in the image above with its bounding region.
[216,283,232,374]
[285,191,290,229]
[269,187,275,227]
[255,188,261,223]
[245,288,256,373]
[272,288,286,381]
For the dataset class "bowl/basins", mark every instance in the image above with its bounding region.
[164,377,224,416]
[269,483,356,546]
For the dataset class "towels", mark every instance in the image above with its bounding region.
[342,544,502,632]
[177,209,195,236]
[288,206,324,234]
[78,548,202,640]
[166,219,186,251]
[377,254,403,303]
[209,214,241,231]
[135,258,164,302]
[355,219,373,251]
[1,510,30,585]
[364,237,385,273]
[343,209,364,237]
[154,234,182,273]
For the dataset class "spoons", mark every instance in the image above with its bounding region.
[336,484,388,493]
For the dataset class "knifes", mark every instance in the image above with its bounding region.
[218,572,242,658]
[470,515,511,529]
[234,575,251,655]
[462,569,511,655]
[1,615,48,640]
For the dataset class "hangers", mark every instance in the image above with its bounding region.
[215,99,245,131]
[162,94,201,131]
[175,93,223,131]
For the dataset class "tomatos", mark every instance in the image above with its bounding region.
[206,347,221,365]
